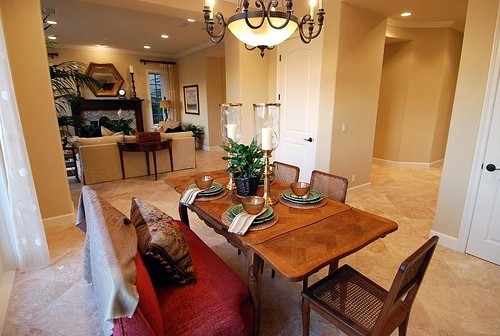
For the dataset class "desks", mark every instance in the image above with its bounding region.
[116,137,174,182]
[71,99,144,136]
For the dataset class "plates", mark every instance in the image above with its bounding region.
[226,204,277,223]
[185,183,225,197]
[281,188,324,204]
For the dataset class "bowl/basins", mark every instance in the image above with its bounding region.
[241,195,265,214]
[194,176,214,190]
[290,182,311,197]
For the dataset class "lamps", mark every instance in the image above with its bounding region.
[129,66,140,100]
[71,62,85,101]
[202,0,326,58]
[159,97,172,121]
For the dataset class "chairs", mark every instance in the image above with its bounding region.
[271,170,347,279]
[300,235,439,336]
[76,186,255,336]
[237,161,300,273]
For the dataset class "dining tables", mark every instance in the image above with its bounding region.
[162,169,398,336]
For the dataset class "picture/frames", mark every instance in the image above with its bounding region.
[183,84,200,115]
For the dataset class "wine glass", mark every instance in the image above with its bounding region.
[253,102,280,205]
[219,102,244,191]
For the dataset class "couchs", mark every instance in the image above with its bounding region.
[75,131,196,185]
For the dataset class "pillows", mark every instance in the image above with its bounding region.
[130,196,196,286]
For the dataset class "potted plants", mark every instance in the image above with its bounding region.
[181,123,204,149]
[218,134,266,199]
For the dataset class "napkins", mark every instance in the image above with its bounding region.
[179,186,214,207]
[227,208,267,236]
